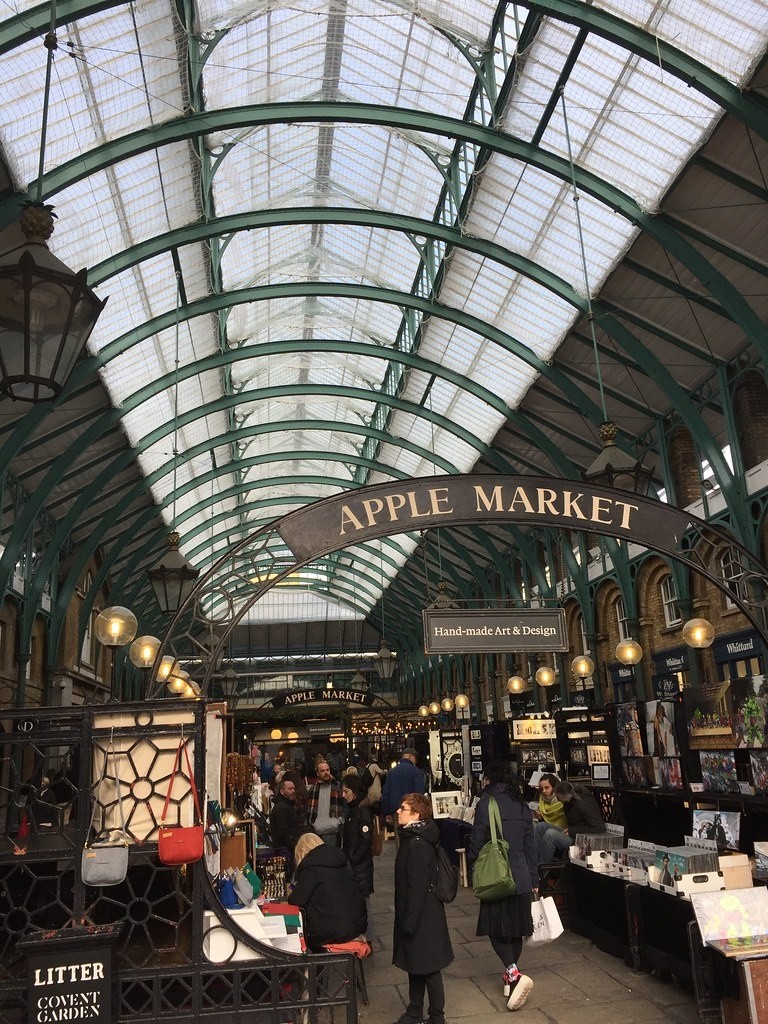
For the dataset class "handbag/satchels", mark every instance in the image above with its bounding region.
[473,840,516,901]
[218,862,262,909]
[524,890,564,948]
[82,847,128,887]
[372,835,382,856]
[158,826,203,865]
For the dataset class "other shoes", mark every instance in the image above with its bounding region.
[392,1013,424,1024]
[424,1008,445,1024]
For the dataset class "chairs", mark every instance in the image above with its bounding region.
[534,786,620,913]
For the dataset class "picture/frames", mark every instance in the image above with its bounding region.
[693,809,742,850]
[608,702,643,756]
[657,757,683,792]
[699,750,740,794]
[730,674,768,747]
[683,681,733,737]
[618,757,656,789]
[749,749,768,795]
[642,698,682,758]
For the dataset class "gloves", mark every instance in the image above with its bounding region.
[397,931,413,942]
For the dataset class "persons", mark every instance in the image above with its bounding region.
[340,773,375,894]
[533,774,607,864]
[653,701,672,757]
[288,833,368,955]
[267,748,425,855]
[466,760,539,1011]
[658,853,671,886]
[389,793,454,1024]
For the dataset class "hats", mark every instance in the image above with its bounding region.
[403,748,420,760]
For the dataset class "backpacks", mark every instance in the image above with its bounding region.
[364,763,377,786]
[433,841,457,903]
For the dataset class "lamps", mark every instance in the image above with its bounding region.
[144,267,202,617]
[197,456,228,672]
[350,560,368,691]
[559,85,657,500]
[0,11,110,405]
[371,537,400,679]
[218,635,241,697]
[222,692,240,712]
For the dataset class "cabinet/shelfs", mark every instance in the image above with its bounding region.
[571,822,768,1024]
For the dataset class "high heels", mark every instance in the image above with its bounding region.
[506,973,534,1010]
[502,973,510,997]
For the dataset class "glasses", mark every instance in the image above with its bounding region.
[401,807,412,812]
